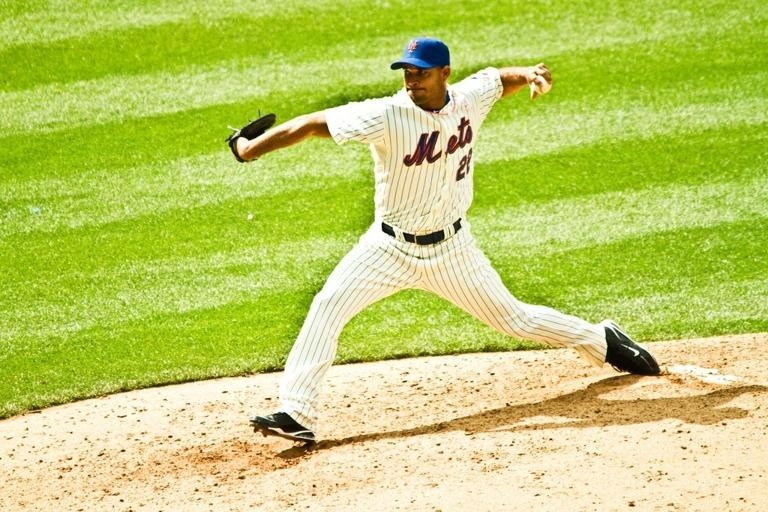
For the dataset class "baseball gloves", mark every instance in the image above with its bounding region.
[228,112,276,163]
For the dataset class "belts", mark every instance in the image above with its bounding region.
[379,216,463,245]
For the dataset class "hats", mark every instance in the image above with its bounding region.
[390,36,451,71]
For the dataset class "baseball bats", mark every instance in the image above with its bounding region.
[531,76,552,95]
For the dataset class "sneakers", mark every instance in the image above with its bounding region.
[248,411,315,444]
[601,317,661,377]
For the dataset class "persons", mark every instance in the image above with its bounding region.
[233,38,660,442]
